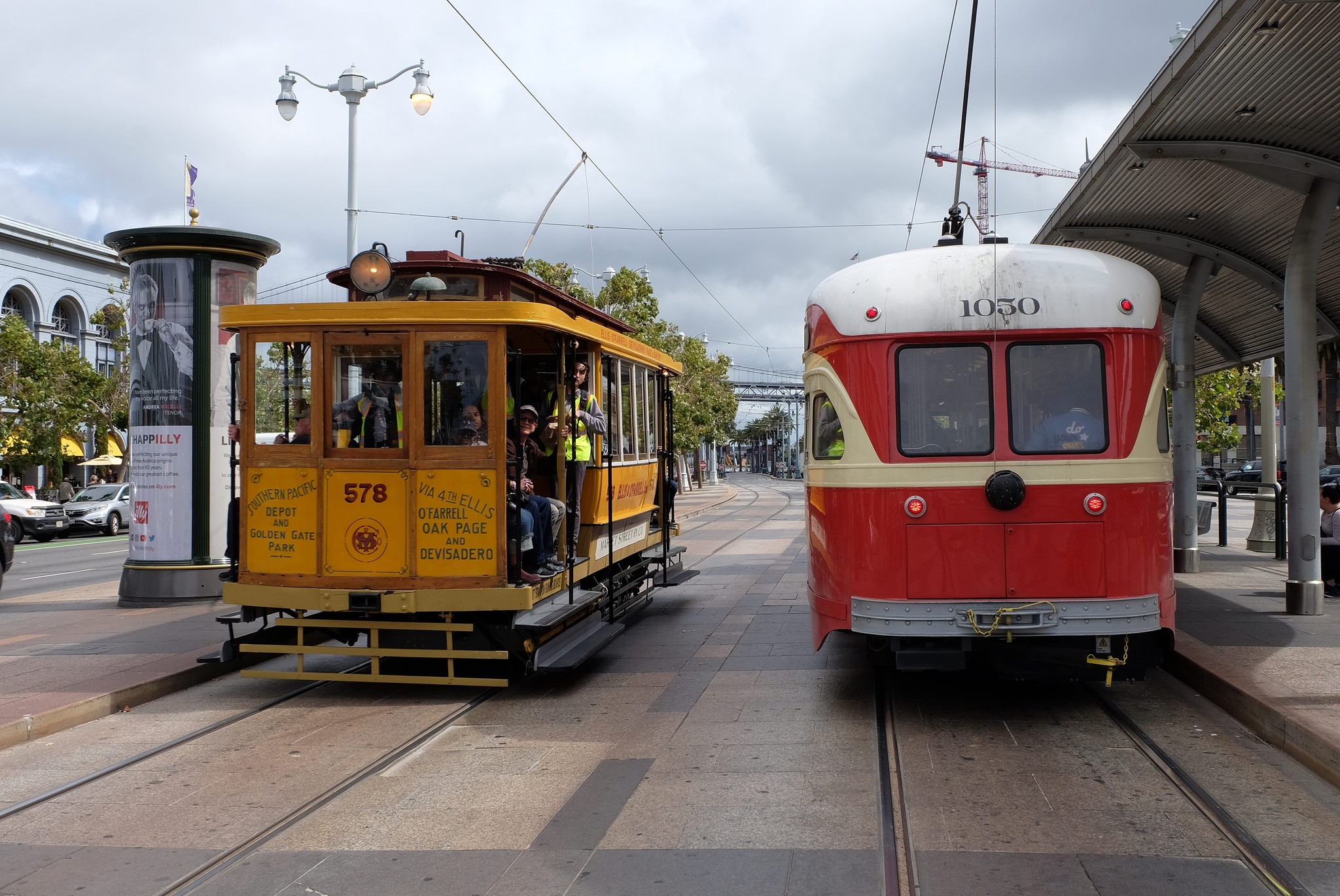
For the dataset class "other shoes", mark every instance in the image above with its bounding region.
[521,567,557,578]
[1324,581,1334,593]
[652,518,678,529]
[546,563,565,572]
[567,549,577,563]
[1324,584,1340,598]
[546,560,564,566]
[509,565,541,583]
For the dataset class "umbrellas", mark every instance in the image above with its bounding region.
[77,454,129,484]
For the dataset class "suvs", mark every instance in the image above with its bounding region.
[1224,459,1288,496]
[60,482,130,536]
[1196,466,1227,491]
[0,479,71,585]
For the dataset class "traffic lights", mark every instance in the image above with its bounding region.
[777,439,782,447]
[772,445,777,451]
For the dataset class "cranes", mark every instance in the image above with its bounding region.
[926,134,1081,246]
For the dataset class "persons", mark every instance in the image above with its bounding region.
[129,274,194,427]
[213,281,256,428]
[651,477,679,529]
[63,474,75,481]
[1023,381,1105,451]
[458,400,557,578]
[536,359,608,564]
[506,405,566,572]
[292,362,402,449]
[273,422,299,445]
[228,408,311,445]
[2,490,11,497]
[1320,483,1340,598]
[470,342,529,436]
[86,475,99,487]
[98,479,106,484]
[105,469,117,484]
[450,415,542,584]
[58,477,76,504]
[1,475,9,482]
[817,398,844,458]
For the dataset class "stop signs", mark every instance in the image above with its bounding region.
[699,460,707,470]
[720,465,724,469]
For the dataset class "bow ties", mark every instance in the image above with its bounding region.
[136,332,153,346]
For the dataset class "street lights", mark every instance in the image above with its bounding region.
[567,262,653,316]
[271,58,435,269]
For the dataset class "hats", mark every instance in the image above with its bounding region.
[521,405,538,418]
[64,477,71,482]
[293,408,310,418]
[456,416,477,433]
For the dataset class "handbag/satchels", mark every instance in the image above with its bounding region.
[509,490,530,509]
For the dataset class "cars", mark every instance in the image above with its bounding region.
[724,467,751,473]
[768,467,772,472]
[1319,464,1340,485]
[784,467,789,473]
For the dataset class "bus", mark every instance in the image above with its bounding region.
[197,147,687,691]
[799,0,1184,690]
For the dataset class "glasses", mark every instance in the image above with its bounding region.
[521,415,537,424]
[459,430,474,436]
